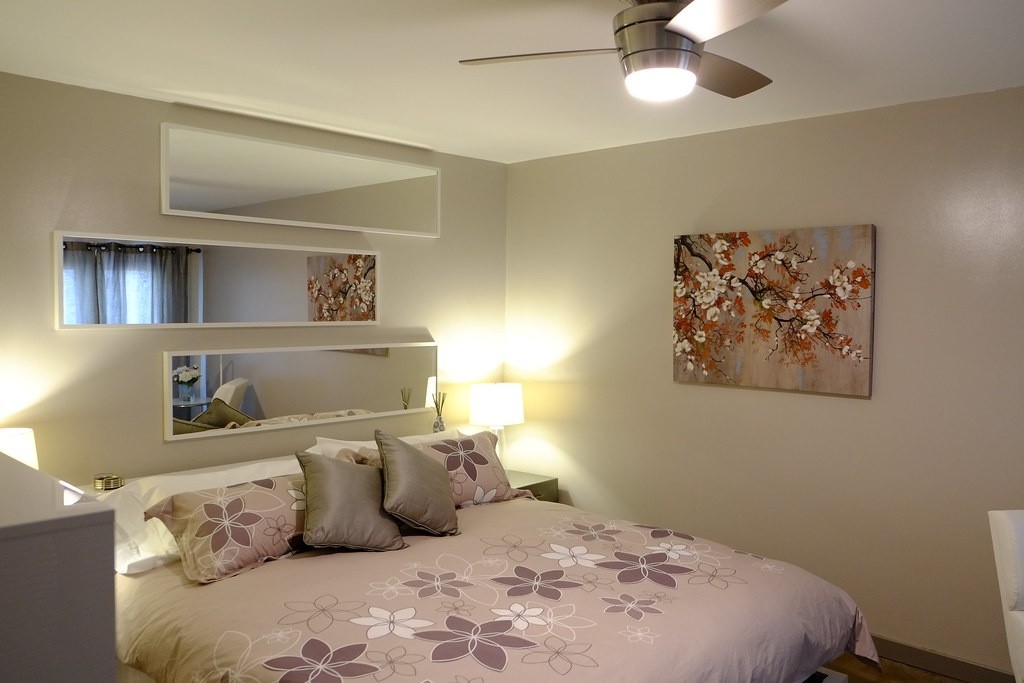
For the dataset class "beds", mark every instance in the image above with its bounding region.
[98,430,884,682]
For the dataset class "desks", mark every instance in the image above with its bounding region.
[173,396,212,418]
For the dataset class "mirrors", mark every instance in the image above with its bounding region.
[161,122,442,239]
[54,232,380,330]
[163,341,440,443]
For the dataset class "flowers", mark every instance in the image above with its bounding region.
[171,365,199,387]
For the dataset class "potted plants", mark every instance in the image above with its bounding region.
[431,392,448,433]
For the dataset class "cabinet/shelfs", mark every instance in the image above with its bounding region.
[0,451,116,682]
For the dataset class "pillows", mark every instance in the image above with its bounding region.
[96,446,320,576]
[357,432,514,521]
[173,417,219,435]
[315,430,461,460]
[144,448,364,586]
[374,428,462,538]
[191,398,256,427]
[295,450,409,553]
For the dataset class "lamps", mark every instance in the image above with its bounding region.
[0,428,40,469]
[619,38,700,100]
[424,376,436,408]
[467,382,525,475]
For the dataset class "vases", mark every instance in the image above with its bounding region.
[181,386,192,402]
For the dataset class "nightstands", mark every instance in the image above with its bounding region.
[504,469,559,503]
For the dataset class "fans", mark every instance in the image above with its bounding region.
[458,1,789,99]
